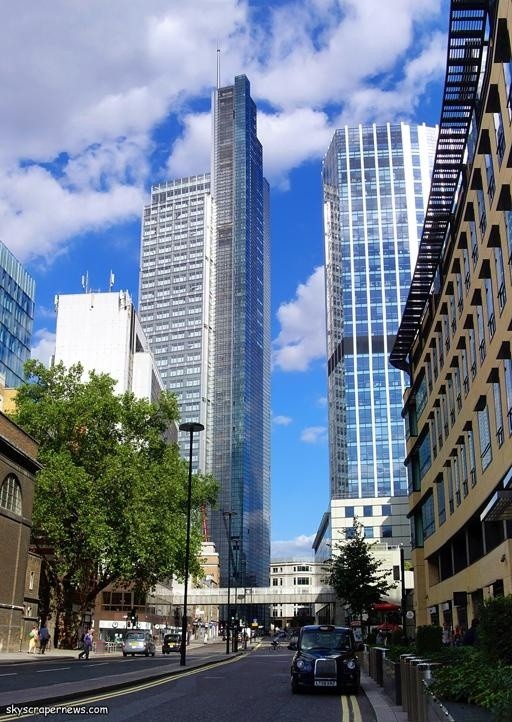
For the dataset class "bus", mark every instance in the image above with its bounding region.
[350,603,405,650]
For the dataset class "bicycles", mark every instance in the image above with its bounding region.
[269,641,284,652]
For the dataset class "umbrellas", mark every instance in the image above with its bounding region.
[370,603,400,637]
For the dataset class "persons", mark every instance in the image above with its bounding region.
[79,628,95,660]
[462,618,480,646]
[242,632,249,649]
[441,621,450,645]
[273,635,280,650]
[376,629,384,644]
[39,623,50,654]
[203,630,209,644]
[28,625,39,654]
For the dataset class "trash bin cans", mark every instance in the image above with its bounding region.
[400,653,443,722]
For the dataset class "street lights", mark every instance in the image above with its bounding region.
[223,511,247,652]
[177,420,204,667]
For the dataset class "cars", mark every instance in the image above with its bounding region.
[161,634,183,655]
[223,626,266,641]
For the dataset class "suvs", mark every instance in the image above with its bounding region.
[287,625,361,695]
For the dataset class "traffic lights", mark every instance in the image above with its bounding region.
[127,621,130,627]
[130,609,137,621]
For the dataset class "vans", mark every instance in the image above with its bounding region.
[123,629,155,658]
